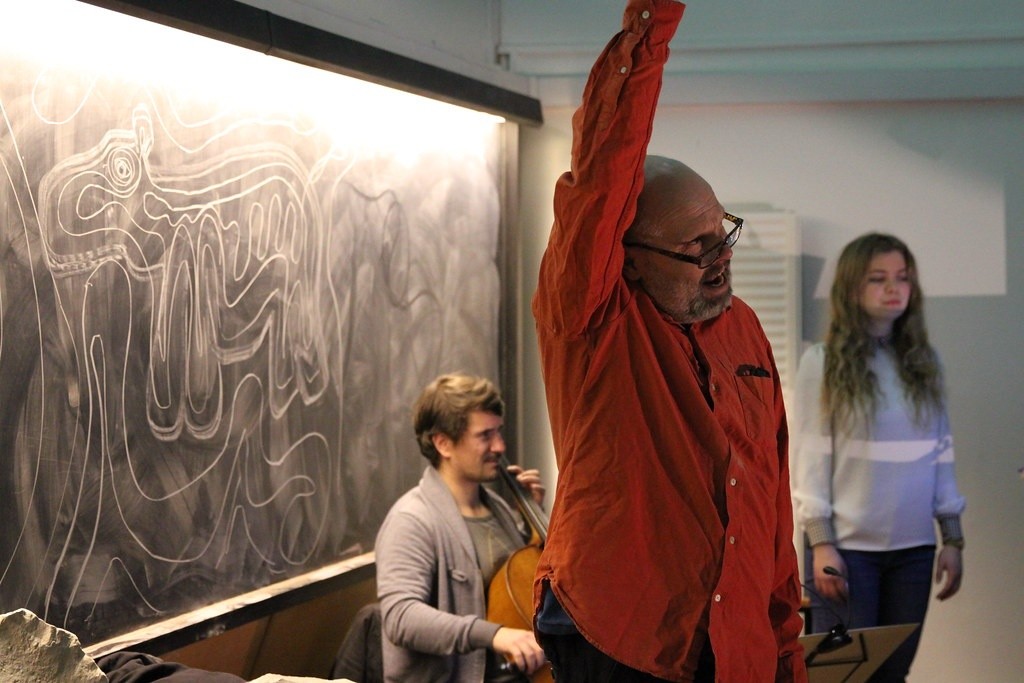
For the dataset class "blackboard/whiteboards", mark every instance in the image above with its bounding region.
[0,0,544,668]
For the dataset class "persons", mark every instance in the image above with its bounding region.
[789,233,965,683]
[374,374,545,683]
[529,0,805,683]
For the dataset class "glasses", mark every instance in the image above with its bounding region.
[622,212,744,268]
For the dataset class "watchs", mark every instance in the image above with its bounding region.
[943,538,965,551]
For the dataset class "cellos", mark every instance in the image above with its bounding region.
[482,454,558,683]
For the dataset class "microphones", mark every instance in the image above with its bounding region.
[821,566,853,633]
